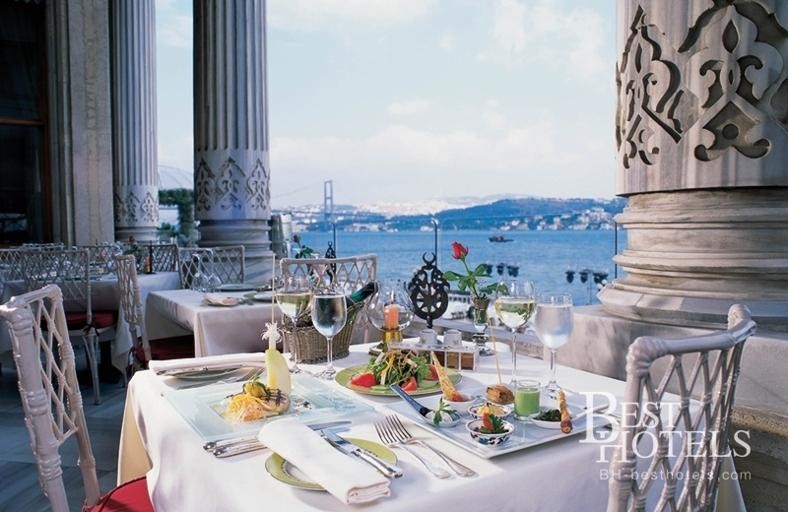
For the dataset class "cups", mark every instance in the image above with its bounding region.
[418,329,462,348]
[514,379,539,422]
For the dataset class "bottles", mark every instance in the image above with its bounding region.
[346,282,377,308]
[366,279,414,332]
[123,236,149,274]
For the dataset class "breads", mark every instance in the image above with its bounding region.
[484,385,515,405]
[230,387,290,421]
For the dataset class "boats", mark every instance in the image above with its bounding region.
[489,234,515,244]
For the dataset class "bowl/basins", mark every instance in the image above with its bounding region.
[442,393,513,446]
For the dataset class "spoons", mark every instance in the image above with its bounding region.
[529,402,612,429]
[388,384,462,427]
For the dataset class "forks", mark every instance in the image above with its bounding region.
[374,423,450,480]
[387,414,475,476]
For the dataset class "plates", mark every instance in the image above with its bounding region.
[243,293,276,303]
[215,283,258,291]
[266,438,397,493]
[334,356,462,397]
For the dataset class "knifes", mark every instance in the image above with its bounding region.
[322,425,403,480]
[202,419,350,458]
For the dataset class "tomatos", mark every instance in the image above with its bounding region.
[402,376,417,391]
[352,373,377,388]
[423,365,438,381]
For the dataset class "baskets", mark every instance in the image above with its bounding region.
[282,293,365,366]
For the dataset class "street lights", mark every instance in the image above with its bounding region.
[565,268,606,305]
[483,262,519,322]
[430,215,440,273]
[596,279,608,294]
[332,215,348,273]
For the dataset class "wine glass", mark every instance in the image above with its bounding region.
[310,294,346,380]
[274,275,311,376]
[495,281,572,395]
[188,252,221,298]
[292,248,334,294]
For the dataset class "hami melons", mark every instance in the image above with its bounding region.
[265,350,291,395]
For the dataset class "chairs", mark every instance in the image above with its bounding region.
[1,236,756,512]
